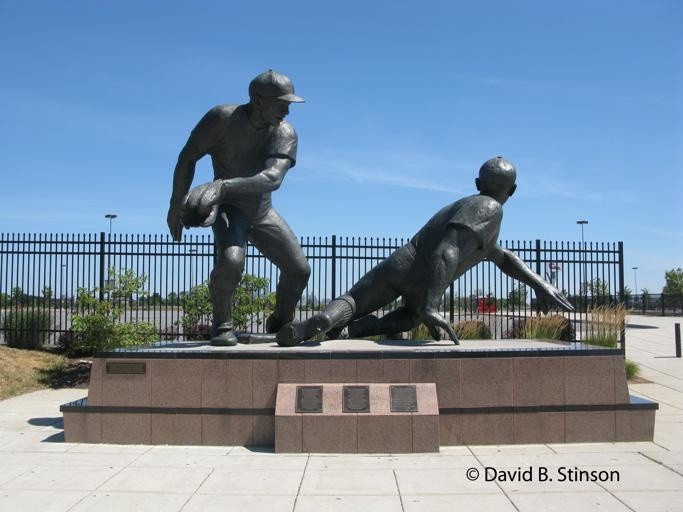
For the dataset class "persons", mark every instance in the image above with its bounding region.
[274,155,575,347]
[164,67,310,347]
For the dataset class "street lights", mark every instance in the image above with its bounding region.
[104,215,117,284]
[631,267,638,301]
[576,221,588,295]
